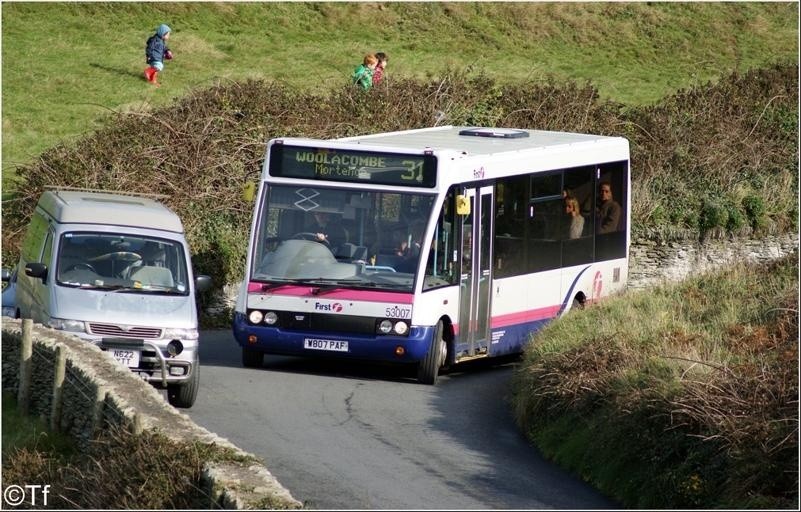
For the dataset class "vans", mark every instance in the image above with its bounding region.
[17,189,211,407]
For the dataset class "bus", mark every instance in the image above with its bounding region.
[233,125,631,385]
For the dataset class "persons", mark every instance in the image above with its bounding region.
[300,211,346,255]
[590,183,622,237]
[374,51,389,84]
[348,55,377,89]
[543,196,585,243]
[559,187,575,199]
[144,23,173,84]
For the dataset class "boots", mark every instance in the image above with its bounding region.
[145,67,159,86]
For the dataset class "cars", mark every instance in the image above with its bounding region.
[2,270,18,318]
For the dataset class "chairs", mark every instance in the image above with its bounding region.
[324,222,351,261]
[61,238,94,273]
[127,248,174,288]
